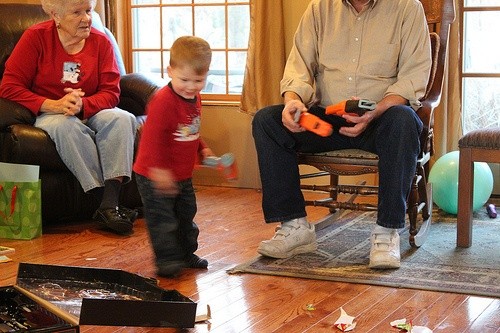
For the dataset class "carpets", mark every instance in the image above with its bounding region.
[226,207,500,298]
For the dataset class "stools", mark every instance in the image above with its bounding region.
[457,125,500,248]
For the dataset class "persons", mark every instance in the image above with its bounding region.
[132,37,219,278]
[251,0,432,269]
[0,0,143,237]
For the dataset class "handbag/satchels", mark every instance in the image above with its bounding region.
[0,179,42,240]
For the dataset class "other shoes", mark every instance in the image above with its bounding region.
[185,256,208,269]
[158,264,179,278]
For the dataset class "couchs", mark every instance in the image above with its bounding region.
[0,4,168,230]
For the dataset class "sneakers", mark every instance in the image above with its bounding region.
[119,207,140,222]
[368,229,402,269]
[92,208,133,233]
[257,224,318,259]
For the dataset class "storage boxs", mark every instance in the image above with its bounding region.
[0,262,198,333]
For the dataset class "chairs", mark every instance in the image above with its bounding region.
[301,1,456,247]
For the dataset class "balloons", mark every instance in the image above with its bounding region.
[428,151,493,216]
